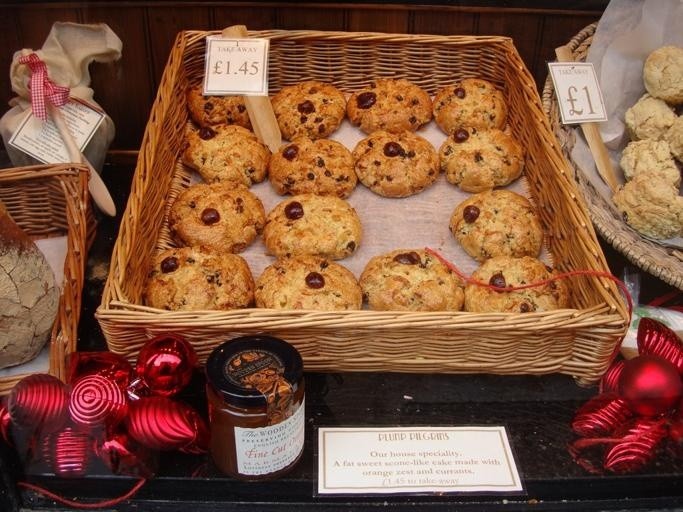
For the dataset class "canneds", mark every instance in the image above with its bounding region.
[204,335,307,484]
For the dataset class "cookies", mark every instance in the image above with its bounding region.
[142,77,570,312]
[613,44,683,243]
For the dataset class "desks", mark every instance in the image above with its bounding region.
[0,143,683,511]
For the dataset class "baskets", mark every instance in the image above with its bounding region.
[542,20,683,292]
[0,163,91,398]
[94,30,632,390]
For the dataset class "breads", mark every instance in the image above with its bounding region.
[0,198,61,370]
